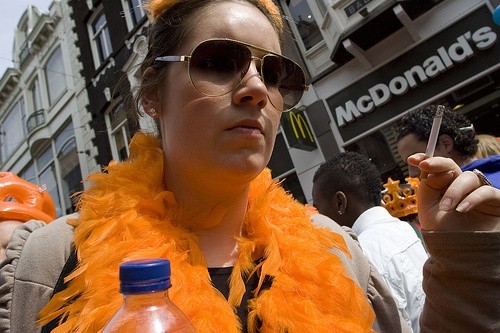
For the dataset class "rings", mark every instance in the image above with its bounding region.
[473,168,493,187]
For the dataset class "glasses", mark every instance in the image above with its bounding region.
[155,38,309,112]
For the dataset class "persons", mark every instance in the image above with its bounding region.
[380,104,500,232]
[311,151,429,332]
[0,0,500,333]
[0,171,56,266]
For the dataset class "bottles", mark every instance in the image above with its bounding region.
[100,259,196,333]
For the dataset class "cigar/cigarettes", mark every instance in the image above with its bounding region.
[418,104,445,182]
